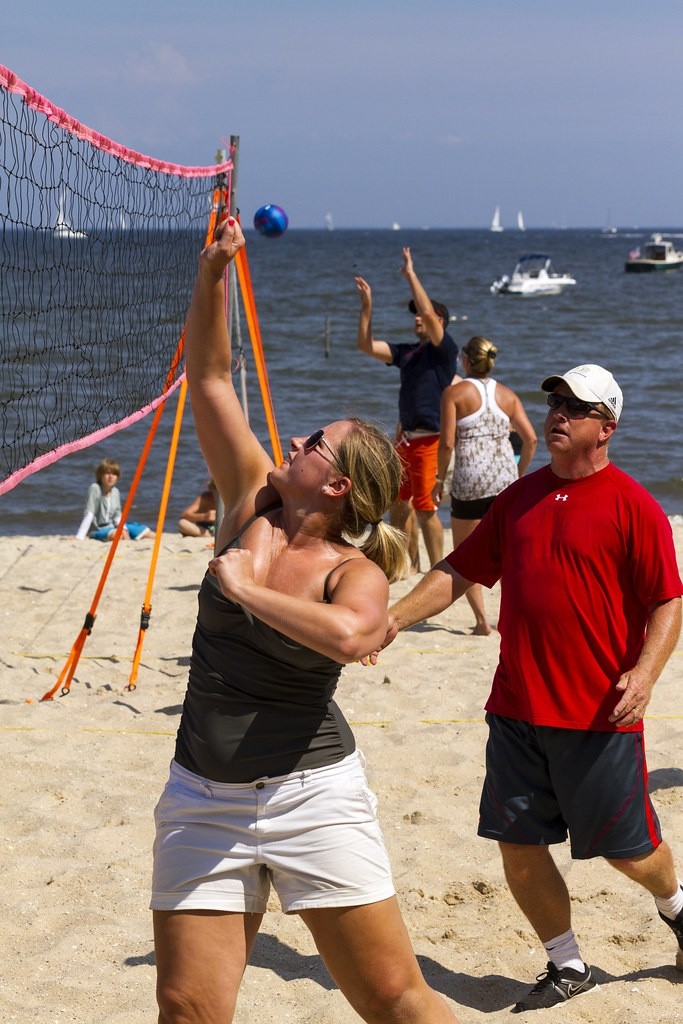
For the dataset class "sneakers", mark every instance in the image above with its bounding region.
[517,961,595,1014]
[657,886,683,953]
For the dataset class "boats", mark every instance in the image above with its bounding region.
[490,254,578,298]
[624,232,683,272]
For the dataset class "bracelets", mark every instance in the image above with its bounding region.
[435,475,446,482]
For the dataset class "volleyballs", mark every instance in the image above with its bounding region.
[254,204,289,239]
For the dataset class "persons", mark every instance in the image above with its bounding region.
[357,364,683,1011]
[179,480,219,537]
[355,247,459,578]
[149,216,458,1024]
[60,457,155,541]
[432,337,538,634]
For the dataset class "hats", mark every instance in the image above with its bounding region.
[409,298,451,329]
[541,364,621,420]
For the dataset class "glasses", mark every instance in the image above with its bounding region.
[302,429,349,474]
[545,393,612,420]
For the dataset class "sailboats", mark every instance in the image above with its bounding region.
[53,188,90,238]
[489,204,505,233]
